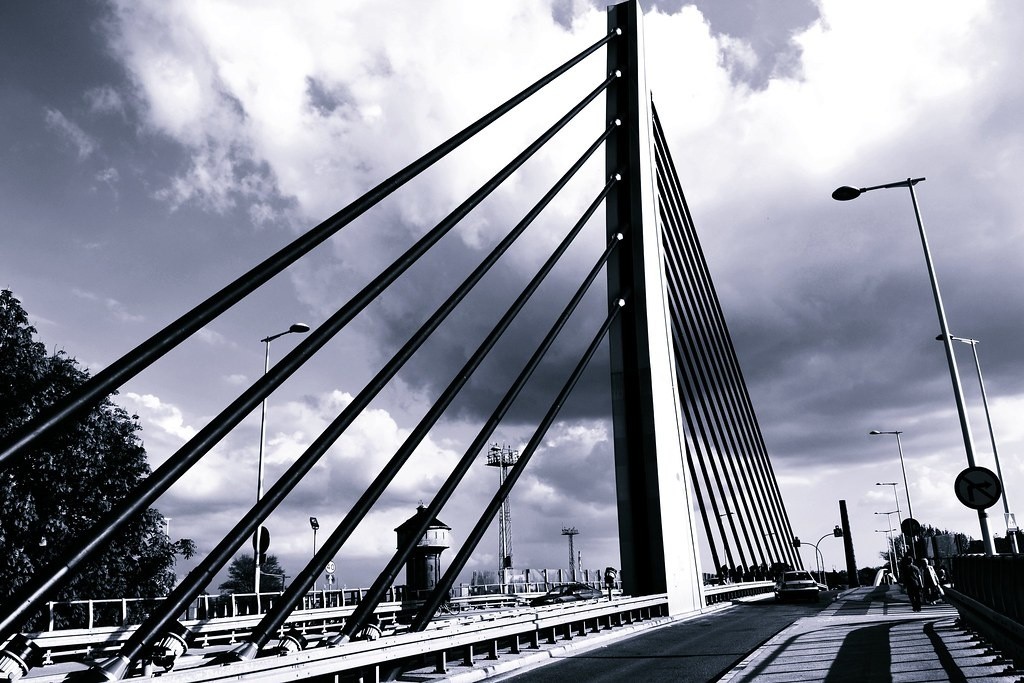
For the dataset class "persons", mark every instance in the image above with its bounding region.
[901,555,946,612]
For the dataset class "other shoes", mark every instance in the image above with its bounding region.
[913,605,921,612]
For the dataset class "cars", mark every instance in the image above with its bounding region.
[529,582,602,607]
[774,570,819,600]
[704,578,730,586]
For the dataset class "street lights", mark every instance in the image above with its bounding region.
[792,526,843,589]
[876,483,910,556]
[308,516,319,608]
[832,175,1001,560]
[253,323,313,599]
[720,512,735,572]
[935,333,1019,553]
[869,431,919,558]
[875,510,902,579]
[876,529,898,579]
